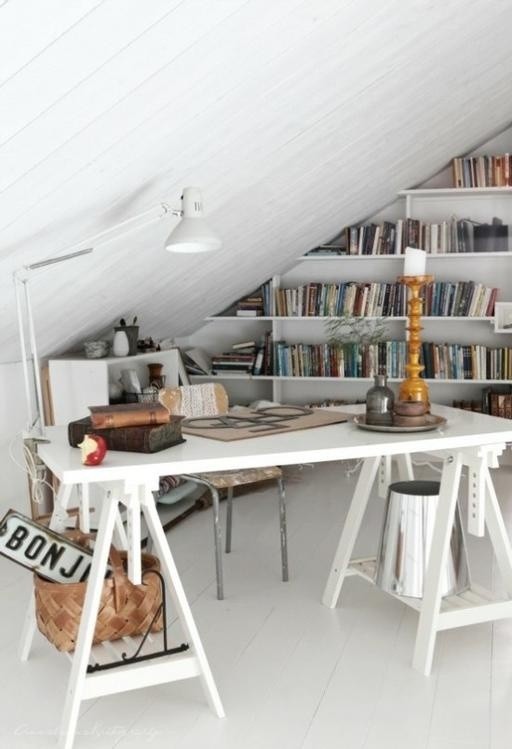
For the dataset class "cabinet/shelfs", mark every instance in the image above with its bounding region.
[48,348,205,548]
[181,185,512,409]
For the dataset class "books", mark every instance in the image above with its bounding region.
[69,415,186,453]
[87,401,170,430]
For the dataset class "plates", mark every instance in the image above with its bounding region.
[353,412,447,433]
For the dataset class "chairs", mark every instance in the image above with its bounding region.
[158,383,290,602]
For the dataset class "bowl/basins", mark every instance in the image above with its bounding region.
[392,416,427,427]
[392,400,427,416]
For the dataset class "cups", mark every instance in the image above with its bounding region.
[83,340,110,360]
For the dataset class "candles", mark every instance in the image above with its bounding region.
[402,245,427,276]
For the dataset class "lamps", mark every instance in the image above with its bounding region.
[12,185,226,446]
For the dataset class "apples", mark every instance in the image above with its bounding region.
[76,433,107,466]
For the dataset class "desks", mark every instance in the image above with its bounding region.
[15,402,511,749]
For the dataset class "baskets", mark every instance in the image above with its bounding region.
[32,529,166,654]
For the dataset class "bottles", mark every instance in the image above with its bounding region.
[112,331,130,357]
[364,374,394,427]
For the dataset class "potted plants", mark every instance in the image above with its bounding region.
[319,302,396,425]
